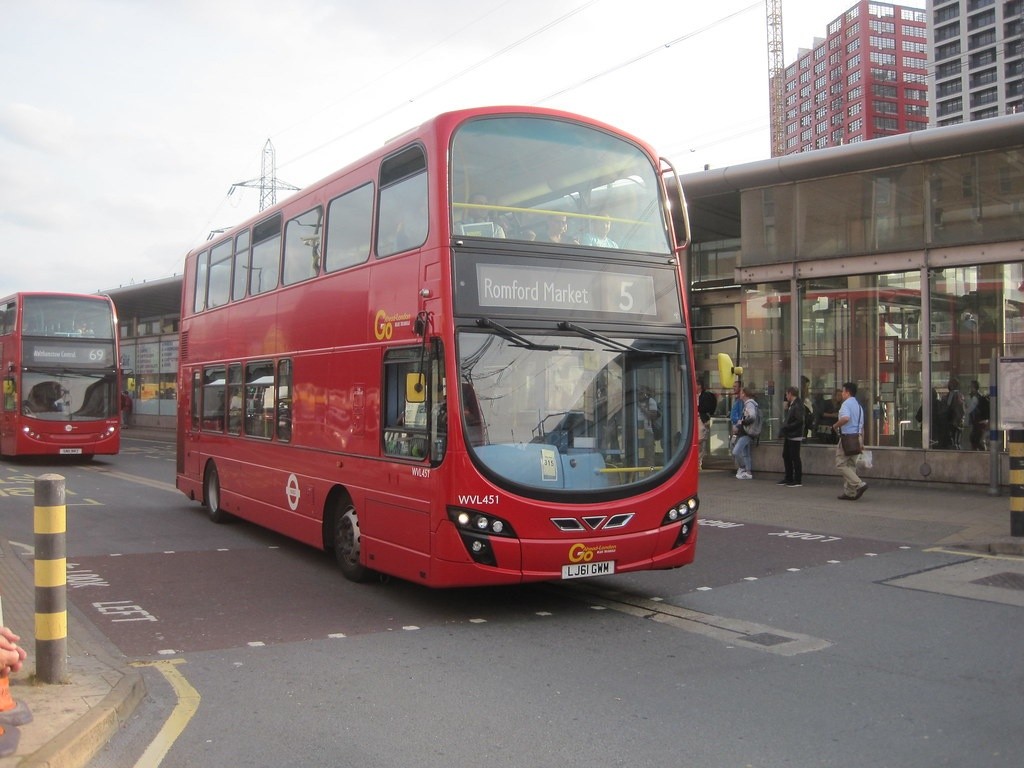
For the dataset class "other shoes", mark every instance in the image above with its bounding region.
[854,482,868,500]
[837,493,855,500]
[777,479,793,485]
[786,481,802,488]
[738,472,752,480]
[736,469,745,478]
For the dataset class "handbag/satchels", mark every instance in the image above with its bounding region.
[645,397,663,441]
[855,449,873,470]
[841,434,862,456]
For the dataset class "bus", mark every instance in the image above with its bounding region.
[175,99,742,589]
[729,282,983,435]
[0,292,135,457]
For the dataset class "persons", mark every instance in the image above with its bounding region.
[695,379,716,470]
[776,386,809,488]
[583,212,619,248]
[725,383,762,479]
[832,383,869,500]
[800,375,843,444]
[535,210,577,243]
[77,322,88,335]
[452,193,506,238]
[121,391,132,429]
[914,379,992,452]
[639,386,658,466]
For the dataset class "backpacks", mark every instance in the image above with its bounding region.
[743,402,763,437]
[976,394,990,420]
